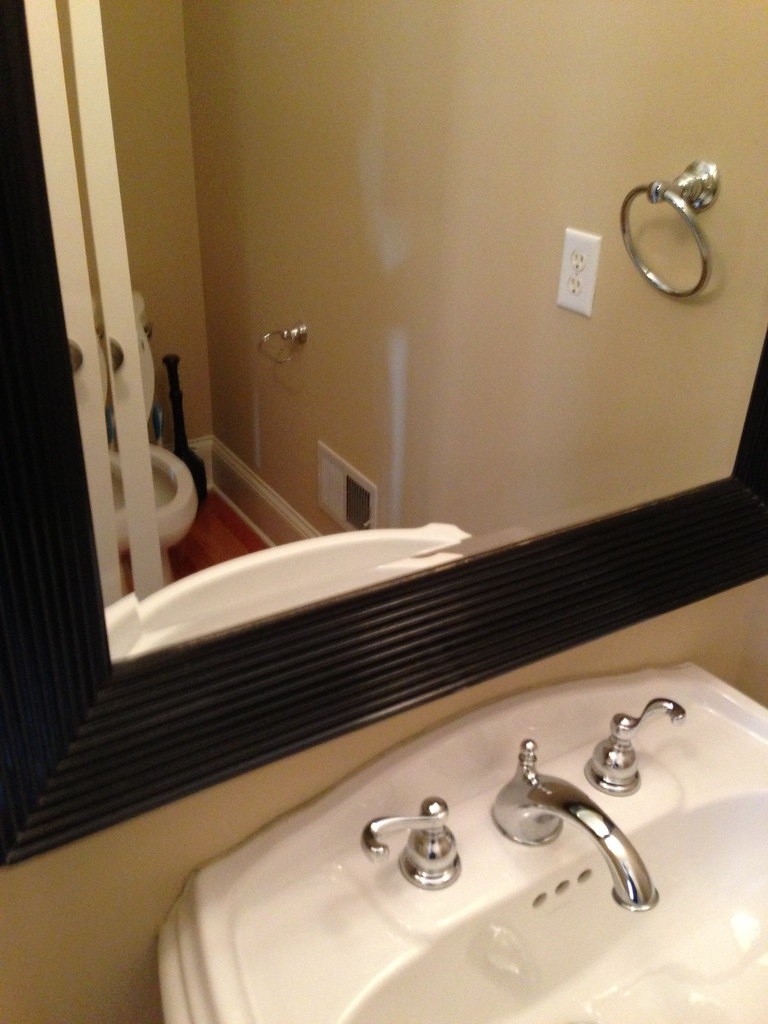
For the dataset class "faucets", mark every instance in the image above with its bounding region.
[488,736,662,911]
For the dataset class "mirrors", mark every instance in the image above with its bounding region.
[60,0,768,598]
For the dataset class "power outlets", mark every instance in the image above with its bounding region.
[557,227,600,313]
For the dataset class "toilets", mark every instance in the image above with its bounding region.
[91,284,202,599]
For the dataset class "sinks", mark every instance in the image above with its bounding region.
[156,662,768,1019]
[117,520,487,653]
